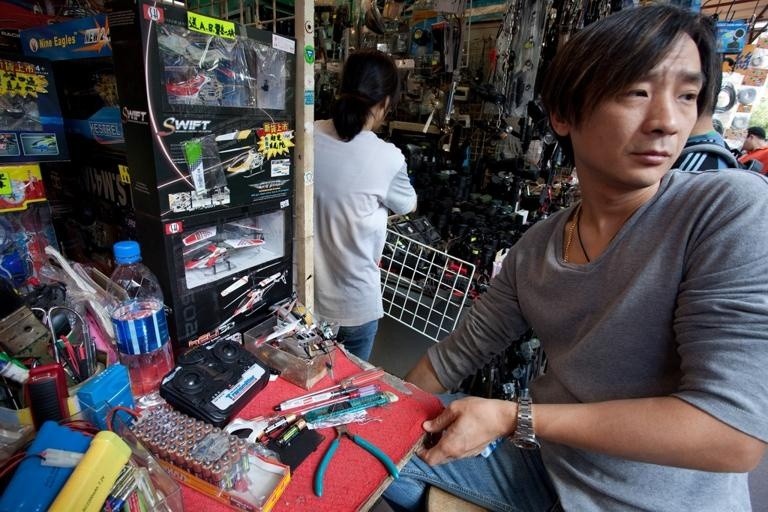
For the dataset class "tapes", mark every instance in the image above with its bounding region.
[67,362,107,397]
[292,306,313,327]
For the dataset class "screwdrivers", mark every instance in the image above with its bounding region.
[283,366,385,416]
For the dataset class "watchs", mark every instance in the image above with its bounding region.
[508,395,541,452]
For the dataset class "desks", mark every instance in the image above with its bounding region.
[71,346,444,512]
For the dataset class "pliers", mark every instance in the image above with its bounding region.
[316,424,399,496]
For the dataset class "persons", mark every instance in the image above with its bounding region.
[669,54,741,175]
[378,3,768,512]
[736,126,768,175]
[311,46,420,361]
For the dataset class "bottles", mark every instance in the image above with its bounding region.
[104,241,175,401]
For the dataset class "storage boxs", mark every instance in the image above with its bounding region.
[0,0,300,351]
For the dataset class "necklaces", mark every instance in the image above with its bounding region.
[563,201,582,265]
[576,207,639,262]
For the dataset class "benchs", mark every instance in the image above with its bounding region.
[427,482,493,512]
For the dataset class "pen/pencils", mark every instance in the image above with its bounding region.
[273,387,360,411]
